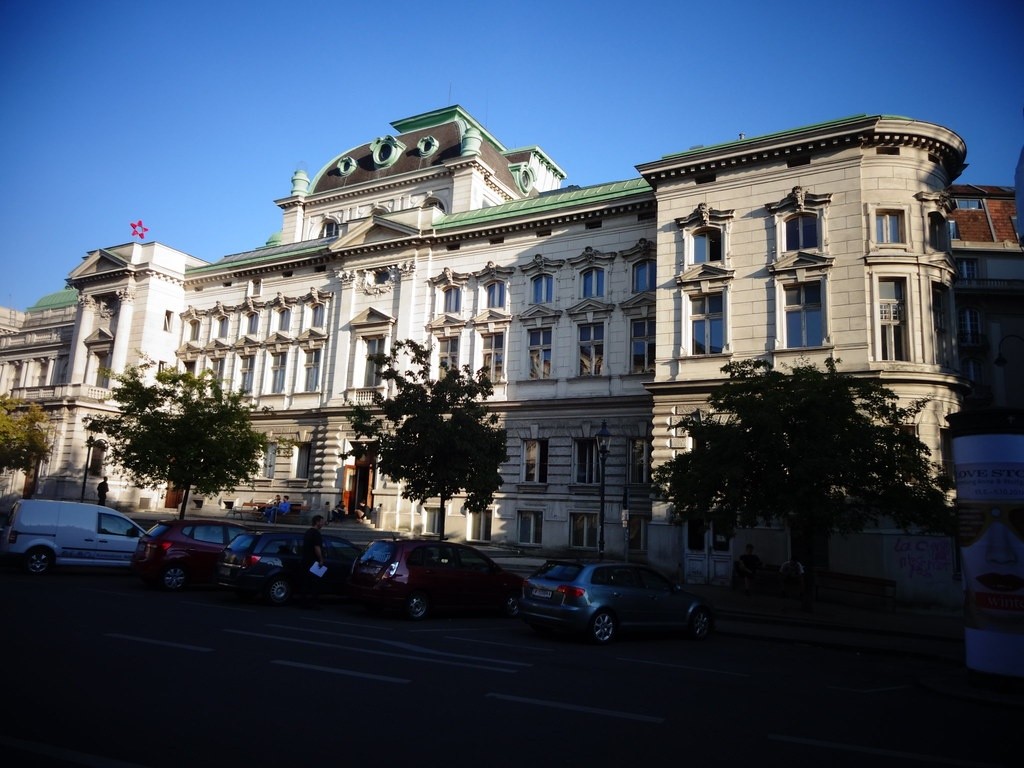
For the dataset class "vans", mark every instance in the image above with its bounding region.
[2,499,152,581]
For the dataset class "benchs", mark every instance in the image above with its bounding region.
[232,509,274,523]
[271,504,305,525]
[732,569,812,613]
[831,571,897,613]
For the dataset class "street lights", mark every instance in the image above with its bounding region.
[79,436,96,503]
[595,418,613,564]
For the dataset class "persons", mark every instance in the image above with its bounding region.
[266,495,290,523]
[332,502,346,521]
[256,495,281,521]
[303,515,325,610]
[779,555,805,598]
[97,477,109,506]
[354,500,371,523]
[737,544,764,592]
[958,502,1024,617]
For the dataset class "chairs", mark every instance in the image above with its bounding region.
[426,551,439,567]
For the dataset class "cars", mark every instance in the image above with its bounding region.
[521,558,717,646]
[218,531,363,607]
[346,530,526,622]
[128,519,258,593]
